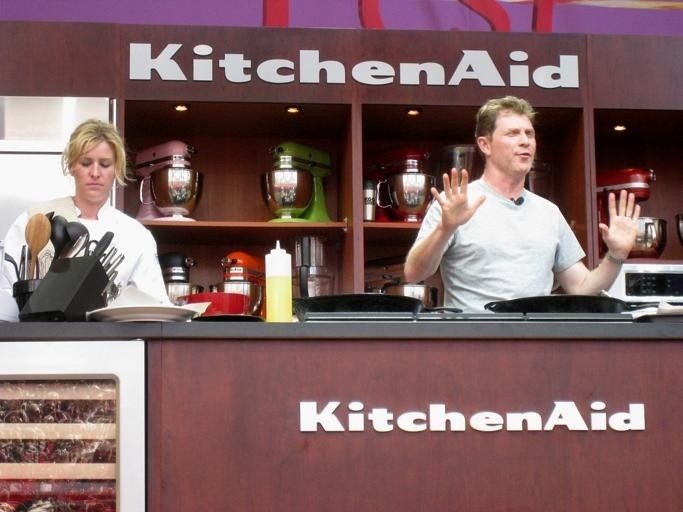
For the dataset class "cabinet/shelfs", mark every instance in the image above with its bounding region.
[126,102,359,305]
[588,110,683,305]
[353,106,591,319]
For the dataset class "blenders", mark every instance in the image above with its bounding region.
[258,140,337,225]
[132,139,203,223]
[589,163,669,261]
[158,247,263,312]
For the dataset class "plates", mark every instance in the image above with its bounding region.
[192,312,264,322]
[84,305,198,324]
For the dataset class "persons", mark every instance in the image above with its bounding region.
[0,119,177,323]
[402,96,640,311]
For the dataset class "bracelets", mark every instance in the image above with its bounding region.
[607,252,628,266]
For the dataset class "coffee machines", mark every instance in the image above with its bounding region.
[293,233,338,302]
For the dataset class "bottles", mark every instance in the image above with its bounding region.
[262,238,292,322]
[364,181,379,222]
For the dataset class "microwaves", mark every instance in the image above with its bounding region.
[597,264,683,303]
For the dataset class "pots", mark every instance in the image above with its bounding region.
[294,293,463,317]
[484,294,683,313]
